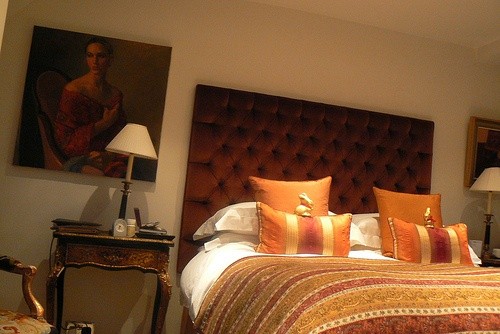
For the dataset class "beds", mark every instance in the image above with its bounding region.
[181,247,500,334]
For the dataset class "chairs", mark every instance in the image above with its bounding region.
[0,256,51,334]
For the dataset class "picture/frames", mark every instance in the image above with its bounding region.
[464,116,500,187]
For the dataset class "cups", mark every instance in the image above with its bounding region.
[492,249,500,258]
[127,225,135,237]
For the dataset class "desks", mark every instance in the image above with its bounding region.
[46,231,175,334]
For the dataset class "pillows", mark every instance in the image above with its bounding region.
[193,175,482,267]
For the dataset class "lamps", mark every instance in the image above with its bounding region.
[468,167,500,259]
[105,123,159,236]
